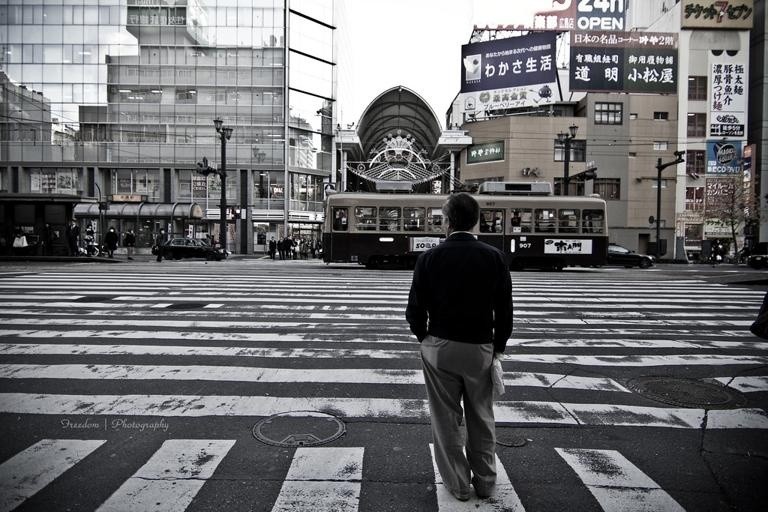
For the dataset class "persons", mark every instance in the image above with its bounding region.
[205,233,210,247]
[266,233,323,261]
[85,223,96,256]
[102,226,118,258]
[125,228,135,260]
[155,228,166,263]
[406,193,516,501]
[208,234,216,247]
[68,217,83,256]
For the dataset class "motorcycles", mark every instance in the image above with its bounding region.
[78,240,108,258]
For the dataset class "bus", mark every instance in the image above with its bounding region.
[320,179,612,276]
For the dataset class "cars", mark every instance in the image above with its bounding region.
[606,241,654,270]
[151,237,229,262]
[747,252,768,270]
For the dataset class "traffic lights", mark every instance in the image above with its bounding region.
[577,172,597,182]
[199,167,218,176]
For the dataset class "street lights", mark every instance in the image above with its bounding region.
[557,122,580,195]
[213,117,234,249]
